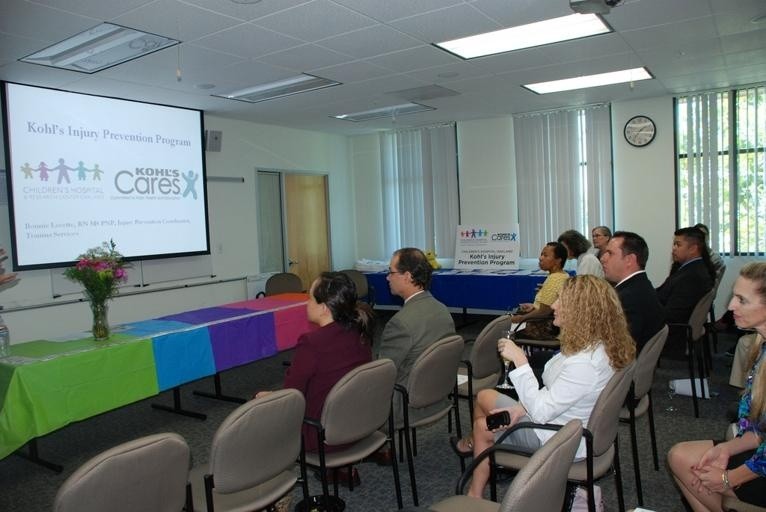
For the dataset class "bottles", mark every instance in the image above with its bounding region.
[0,314,9,359]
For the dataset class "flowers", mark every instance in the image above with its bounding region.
[60,238,135,337]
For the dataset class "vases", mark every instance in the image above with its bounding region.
[0,310,9,358]
[82,288,114,343]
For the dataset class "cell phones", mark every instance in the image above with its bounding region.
[485,411,510,431]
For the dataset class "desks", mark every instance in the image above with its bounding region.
[361,265,576,334]
[0,292,320,476]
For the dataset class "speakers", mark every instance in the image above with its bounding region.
[206,129,222,152]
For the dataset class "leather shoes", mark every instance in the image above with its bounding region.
[366,448,390,465]
[313,467,361,487]
[450,435,474,458]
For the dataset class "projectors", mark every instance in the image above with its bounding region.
[569,0,610,14]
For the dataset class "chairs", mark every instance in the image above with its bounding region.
[487,358,639,512]
[658,288,717,418]
[708,263,728,371]
[296,358,405,512]
[338,269,376,348]
[618,324,669,506]
[51,432,198,512]
[189,388,311,511]
[395,334,467,507]
[255,272,307,299]
[446,313,512,436]
[426,418,584,512]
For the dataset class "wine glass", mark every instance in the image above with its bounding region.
[664,381,680,412]
[496,329,516,389]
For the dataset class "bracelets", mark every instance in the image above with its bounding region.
[721,468,731,490]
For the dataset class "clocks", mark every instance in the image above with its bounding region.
[623,115,656,148]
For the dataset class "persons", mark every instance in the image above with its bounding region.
[694,223,725,268]
[502,225,613,345]
[727,332,759,421]
[449,274,637,499]
[254,273,373,484]
[654,226,714,347]
[339,247,457,486]
[599,231,666,410]
[703,311,744,357]
[668,261,766,511]
